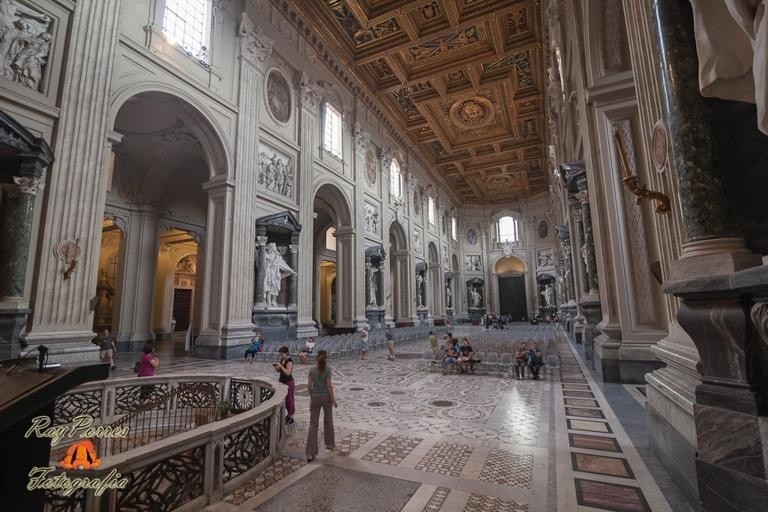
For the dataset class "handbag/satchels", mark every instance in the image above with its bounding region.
[134,361,141,372]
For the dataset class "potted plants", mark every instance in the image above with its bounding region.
[216,400,233,418]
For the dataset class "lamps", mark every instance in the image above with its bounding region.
[608,123,671,214]
[62,238,80,281]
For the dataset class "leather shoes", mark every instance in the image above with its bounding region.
[327,442,336,450]
[308,455,315,463]
[286,415,290,421]
[286,417,294,424]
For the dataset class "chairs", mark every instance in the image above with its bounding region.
[419,321,560,380]
[242,325,454,364]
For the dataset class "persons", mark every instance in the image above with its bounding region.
[554,315,560,335]
[134,342,158,399]
[416,271,424,308]
[480,311,513,330]
[545,316,550,327]
[471,287,481,307]
[527,339,541,380]
[429,330,478,373]
[98,329,117,371]
[530,317,539,325]
[446,283,452,308]
[304,350,338,462]
[361,324,369,360]
[272,346,294,424]
[514,340,529,379]
[171,316,177,331]
[298,335,315,363]
[264,242,295,306]
[385,324,392,338]
[244,333,264,360]
[385,327,395,361]
[366,263,382,307]
[541,284,550,307]
